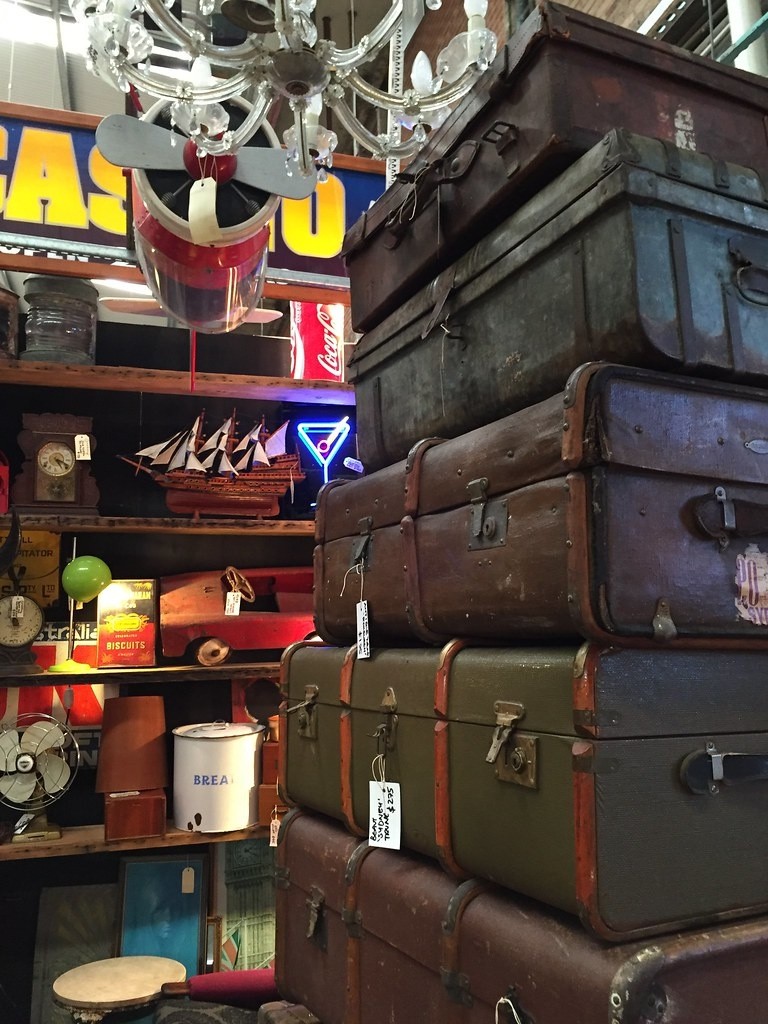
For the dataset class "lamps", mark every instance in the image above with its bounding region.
[64,1,497,181]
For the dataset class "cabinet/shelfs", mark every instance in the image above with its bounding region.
[0,252,352,862]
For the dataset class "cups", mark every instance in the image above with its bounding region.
[94,697,169,792]
[172,723,266,832]
[268,715,278,741]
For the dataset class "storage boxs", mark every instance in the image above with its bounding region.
[274,300,345,382]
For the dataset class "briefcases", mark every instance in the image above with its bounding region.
[275,0,768,1024]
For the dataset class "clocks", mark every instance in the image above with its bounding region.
[9,412,99,515]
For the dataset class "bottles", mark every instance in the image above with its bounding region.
[21,274,100,364]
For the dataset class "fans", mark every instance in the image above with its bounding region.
[0,713,80,843]
